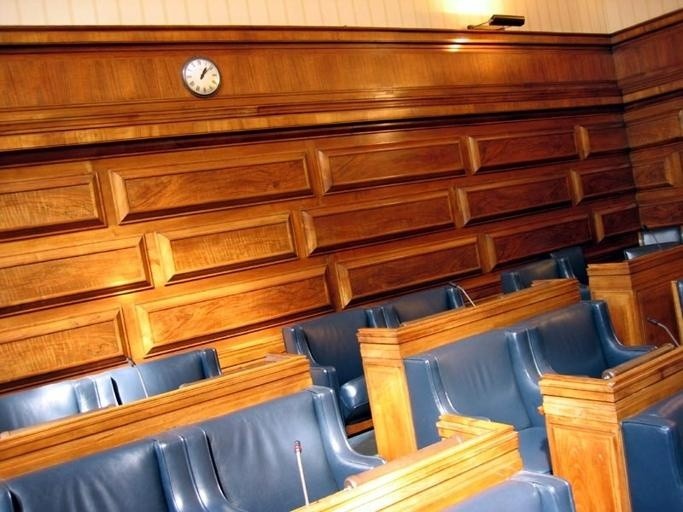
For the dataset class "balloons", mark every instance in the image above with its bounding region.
[181,55,221,97]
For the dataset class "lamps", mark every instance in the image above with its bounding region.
[467,13,525,33]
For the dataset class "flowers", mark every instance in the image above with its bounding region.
[647,317,680,347]
[448,281,475,306]
[126,357,149,398]
[643,224,662,250]
[295,440,310,505]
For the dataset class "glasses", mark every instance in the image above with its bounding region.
[467,13,525,33]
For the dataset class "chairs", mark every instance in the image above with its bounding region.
[283,225,683,512]
[1,348,575,511]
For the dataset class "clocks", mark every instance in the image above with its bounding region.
[181,55,221,97]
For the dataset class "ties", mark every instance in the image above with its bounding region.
[623,242,678,259]
